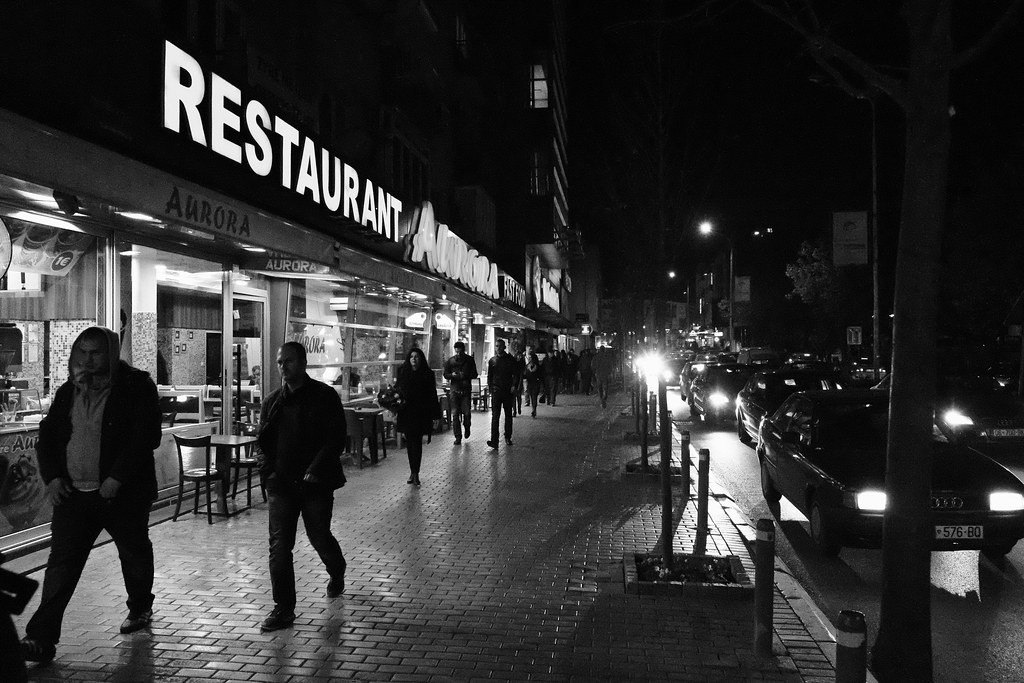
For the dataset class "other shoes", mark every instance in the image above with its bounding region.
[407,474,421,485]
[504,439,513,445]
[260,607,296,630]
[525,403,530,406]
[19,637,56,662]
[547,401,556,407]
[465,427,470,439]
[602,397,607,409]
[120,609,153,634]
[324,559,346,597]
[454,439,461,444]
[531,411,536,418]
[487,440,499,450]
[513,409,521,417]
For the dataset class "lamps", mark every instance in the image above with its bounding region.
[52,190,79,217]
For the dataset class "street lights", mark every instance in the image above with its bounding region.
[670,271,691,335]
[699,221,735,341]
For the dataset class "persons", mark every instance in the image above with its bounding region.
[394,347,441,485]
[247,365,260,385]
[258,342,348,632]
[333,366,361,386]
[513,350,526,416]
[536,345,616,409]
[444,342,476,443]
[487,338,518,449]
[15,327,163,661]
[524,351,542,417]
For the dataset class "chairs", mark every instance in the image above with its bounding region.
[232,377,487,507]
[172,434,217,524]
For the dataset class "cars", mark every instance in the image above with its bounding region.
[615,337,1024,575]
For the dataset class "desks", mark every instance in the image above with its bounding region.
[342,406,383,456]
[208,434,258,519]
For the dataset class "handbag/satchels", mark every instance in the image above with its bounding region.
[377,382,407,414]
